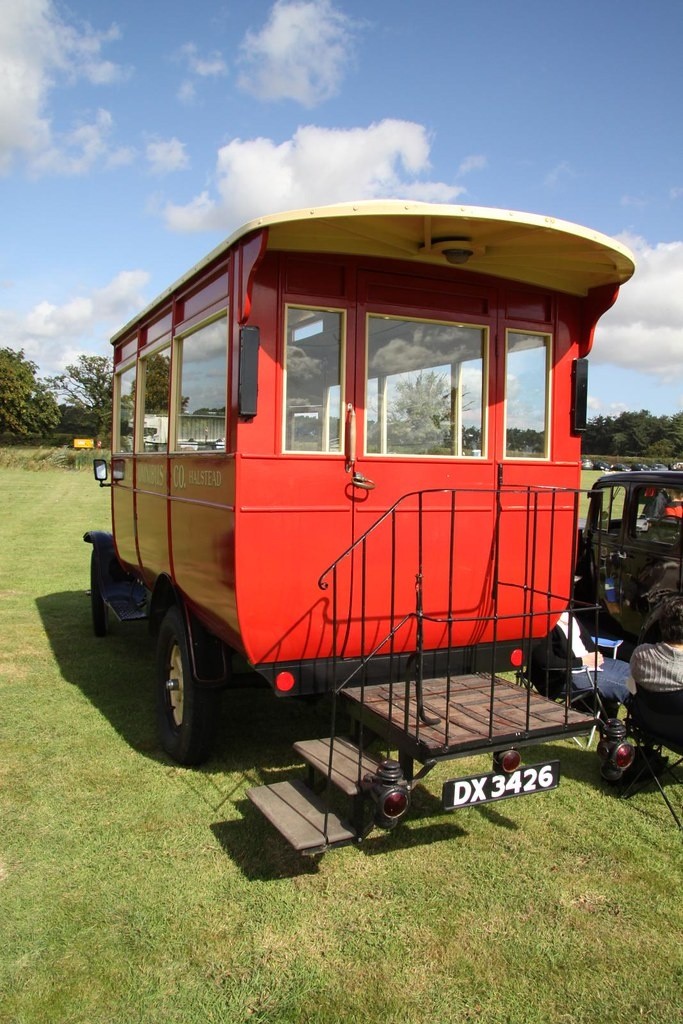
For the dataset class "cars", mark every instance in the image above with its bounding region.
[581,458,683,472]
[529,468,683,653]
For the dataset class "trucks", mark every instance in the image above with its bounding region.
[126,414,225,452]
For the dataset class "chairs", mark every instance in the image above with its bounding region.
[619,674,683,831]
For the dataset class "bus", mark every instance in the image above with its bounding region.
[84,203,631,857]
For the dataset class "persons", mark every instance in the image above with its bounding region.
[665,488,683,518]
[527,611,619,720]
[630,597,683,692]
[640,488,668,518]
